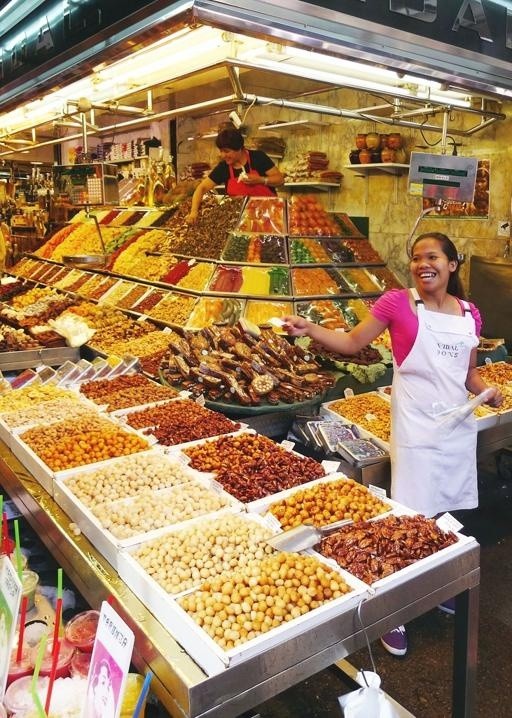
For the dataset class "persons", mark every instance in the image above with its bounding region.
[279,233,504,657]
[185,128,285,226]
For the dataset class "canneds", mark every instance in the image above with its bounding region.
[349,149,359,163]
[388,132,402,149]
[356,134,366,148]
[365,132,379,149]
[378,134,390,147]
[360,151,373,163]
[396,148,408,164]
[371,151,381,163]
[381,148,397,163]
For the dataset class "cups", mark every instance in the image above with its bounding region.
[0,536,149,718]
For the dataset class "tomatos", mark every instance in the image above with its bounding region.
[289,193,340,237]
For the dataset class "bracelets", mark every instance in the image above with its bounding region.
[265,176,268,186]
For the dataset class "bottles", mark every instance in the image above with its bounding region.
[5,243,21,270]
[26,185,50,211]
[145,146,176,208]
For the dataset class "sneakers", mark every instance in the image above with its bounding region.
[438,597,456,614]
[381,625,407,655]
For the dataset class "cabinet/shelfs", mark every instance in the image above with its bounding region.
[317,422,511,493]
[1,347,82,372]
[1,437,482,718]
